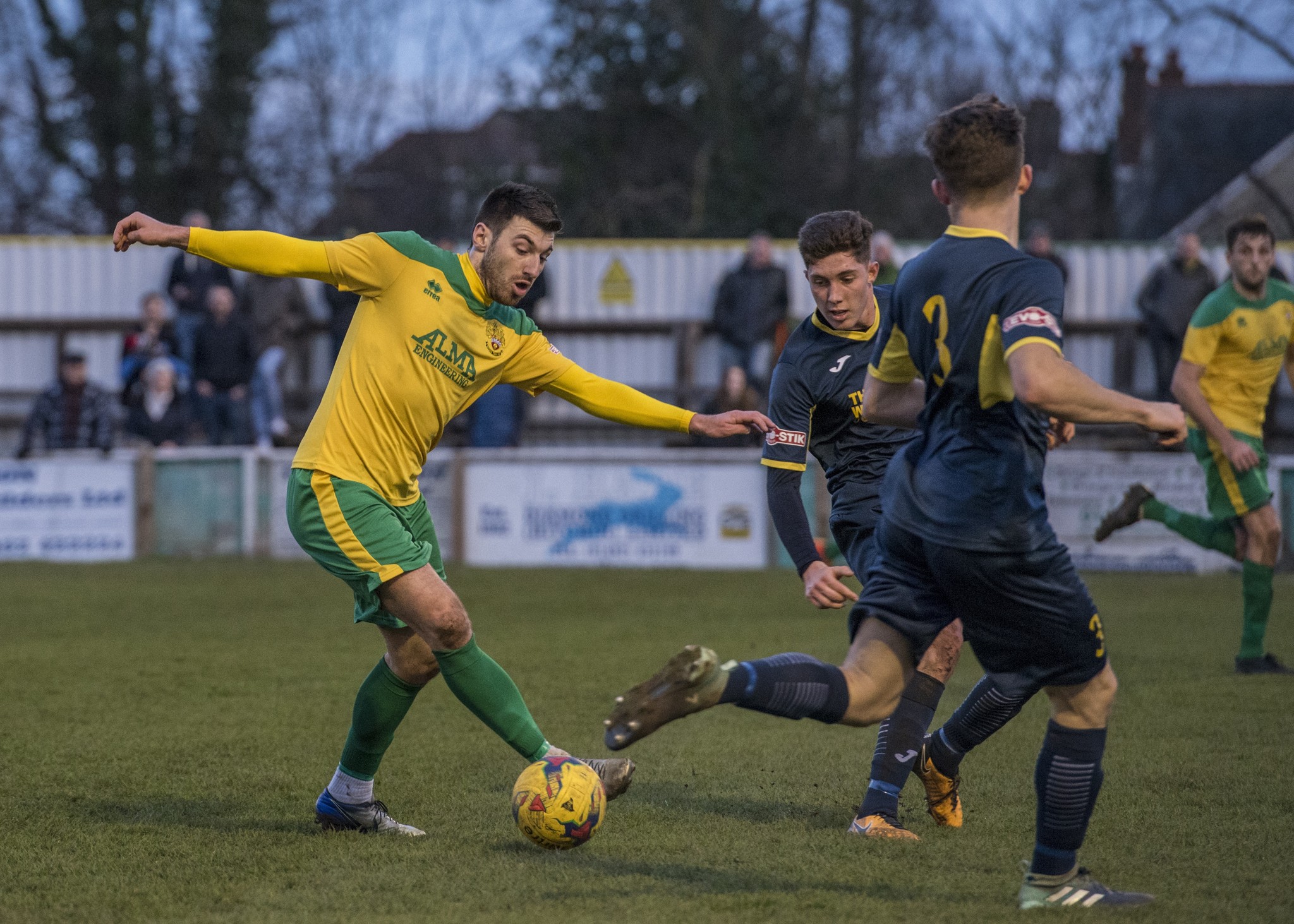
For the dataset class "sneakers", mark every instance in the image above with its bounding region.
[314,788,427,838]
[1017,870,1154,908]
[848,812,919,839]
[579,757,635,800]
[1096,485,1152,542]
[603,645,732,752]
[1235,655,1291,674]
[913,733,964,827]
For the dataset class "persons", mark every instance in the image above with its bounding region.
[463,383,523,446]
[122,294,196,382]
[191,286,259,442]
[242,273,311,449]
[1095,214,1294,675]
[124,357,200,446]
[323,228,363,361]
[111,178,780,838]
[168,210,238,340]
[1023,219,1069,285]
[1139,234,1219,404]
[867,231,902,288]
[17,349,112,458]
[762,210,1043,847]
[701,366,765,447]
[598,91,1154,910]
[708,233,787,398]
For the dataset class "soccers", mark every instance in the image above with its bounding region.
[511,755,606,853]
